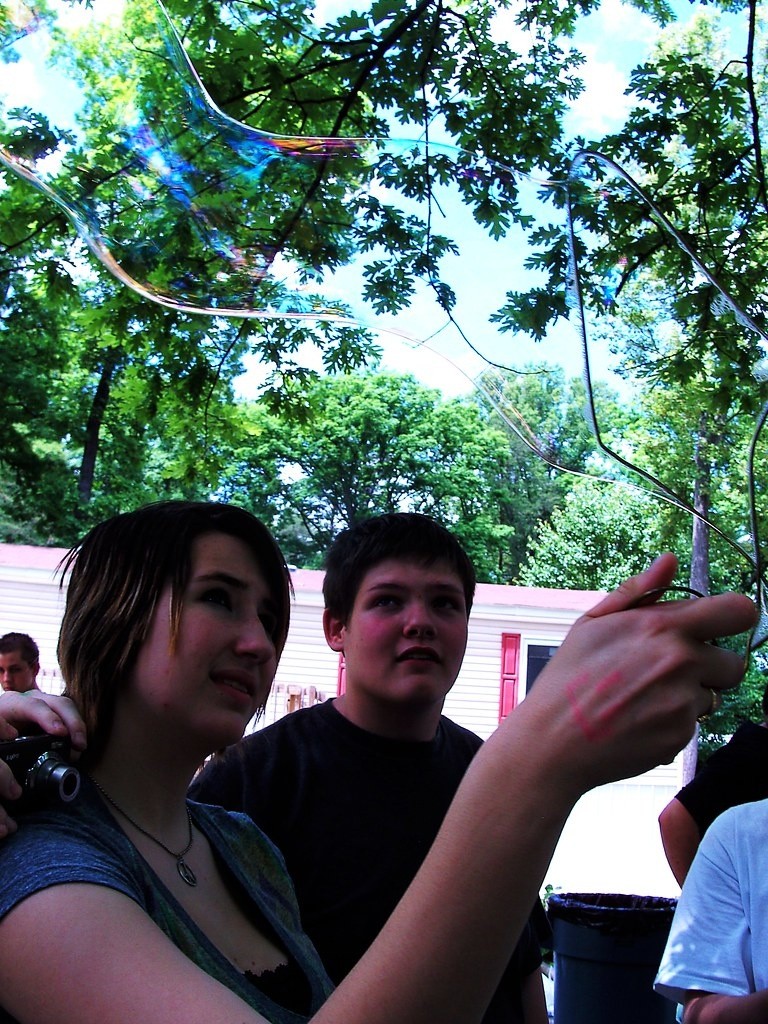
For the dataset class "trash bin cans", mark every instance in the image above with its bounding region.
[547,892,681,1024]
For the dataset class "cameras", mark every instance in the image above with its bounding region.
[0,733,81,828]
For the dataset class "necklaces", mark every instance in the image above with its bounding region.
[88,776,198,888]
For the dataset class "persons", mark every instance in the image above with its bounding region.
[0,500,768,1024]
